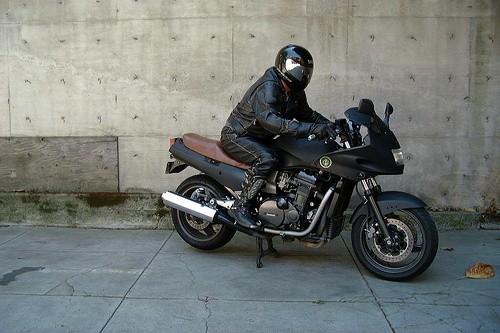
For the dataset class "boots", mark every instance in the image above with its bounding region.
[228,168,266,228]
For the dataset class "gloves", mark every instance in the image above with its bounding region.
[324,123,343,140]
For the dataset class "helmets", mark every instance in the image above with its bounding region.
[274,44,313,91]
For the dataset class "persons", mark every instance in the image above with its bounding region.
[221,43,362,232]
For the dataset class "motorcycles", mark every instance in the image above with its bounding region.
[160,97,442,282]
